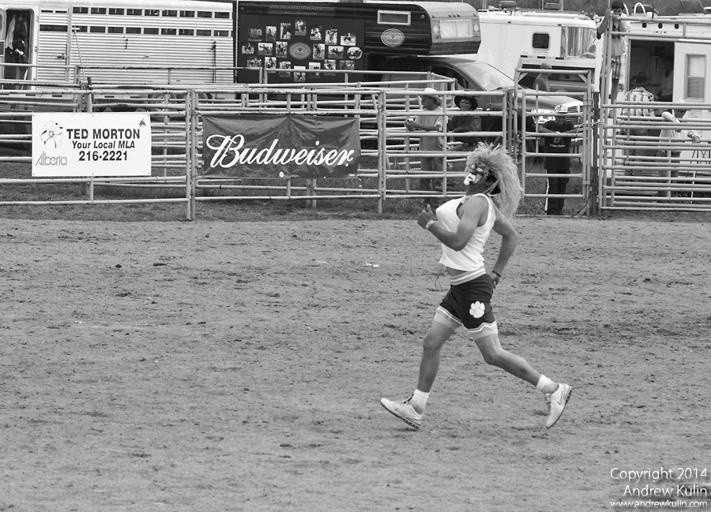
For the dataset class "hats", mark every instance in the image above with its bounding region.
[424,88,442,104]
[554,104,568,112]
[454,96,478,110]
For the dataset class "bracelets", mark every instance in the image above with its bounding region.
[425,218,441,232]
[491,268,503,279]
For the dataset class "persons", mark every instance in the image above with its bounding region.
[596,1,634,112]
[240,17,362,83]
[617,70,657,183]
[536,104,577,216]
[532,62,558,164]
[447,96,486,199]
[403,87,449,207]
[655,53,674,103]
[374,139,576,436]
[656,100,705,203]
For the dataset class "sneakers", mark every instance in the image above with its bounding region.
[545,382,572,429]
[380,394,423,430]
